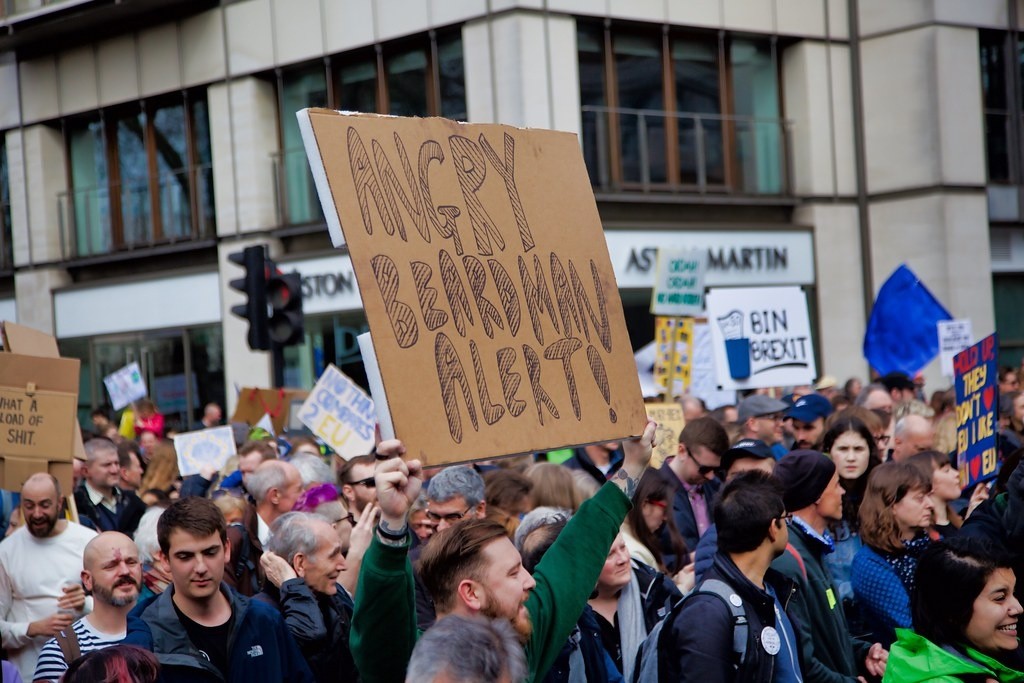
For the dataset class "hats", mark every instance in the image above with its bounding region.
[779,392,804,404]
[133,413,165,438]
[881,372,915,391]
[730,437,771,458]
[815,375,837,389]
[736,394,790,423]
[770,443,789,461]
[783,393,834,423]
[770,450,837,511]
[90,402,112,420]
[291,483,343,513]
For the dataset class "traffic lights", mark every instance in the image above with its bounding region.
[268,272,306,350]
[226,246,268,351]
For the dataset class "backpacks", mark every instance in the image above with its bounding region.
[631,580,746,683]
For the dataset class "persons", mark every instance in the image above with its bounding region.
[657,469,812,683]
[34,531,144,683]
[250,509,354,682]
[879,535,1024,683]
[116,495,317,683]
[0,471,102,683]
[0,372,1024,683]
[350,422,664,682]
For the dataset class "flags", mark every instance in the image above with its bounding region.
[862,263,955,378]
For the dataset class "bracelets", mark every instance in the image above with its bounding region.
[377,523,410,539]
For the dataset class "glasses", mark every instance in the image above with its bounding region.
[760,414,789,421]
[775,512,793,529]
[871,408,892,416]
[873,434,891,445]
[346,476,376,488]
[509,510,527,522]
[223,518,244,528]
[425,505,474,524]
[685,447,722,476]
[644,498,669,513]
